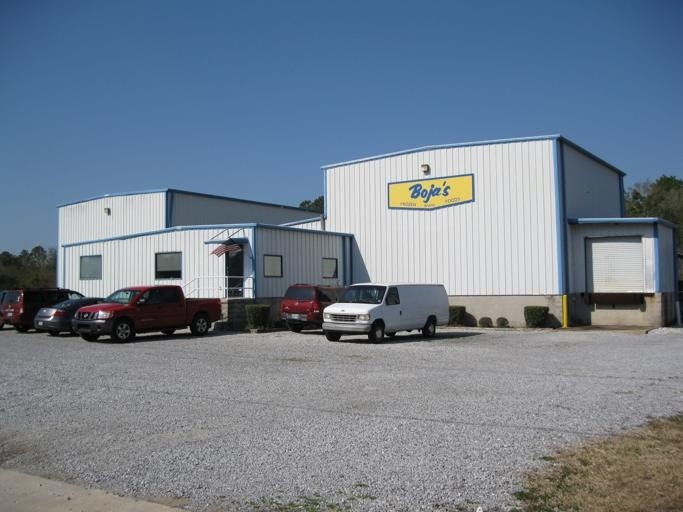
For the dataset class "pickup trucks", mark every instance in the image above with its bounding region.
[71,285,222,341]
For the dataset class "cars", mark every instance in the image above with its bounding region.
[33,298,105,336]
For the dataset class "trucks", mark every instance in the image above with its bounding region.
[322,282,450,344]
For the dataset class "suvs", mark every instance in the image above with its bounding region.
[0,287,85,332]
[279,283,349,332]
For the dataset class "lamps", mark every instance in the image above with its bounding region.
[421,165,428,172]
[105,209,110,215]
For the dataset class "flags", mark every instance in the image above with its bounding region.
[210,239,242,258]
